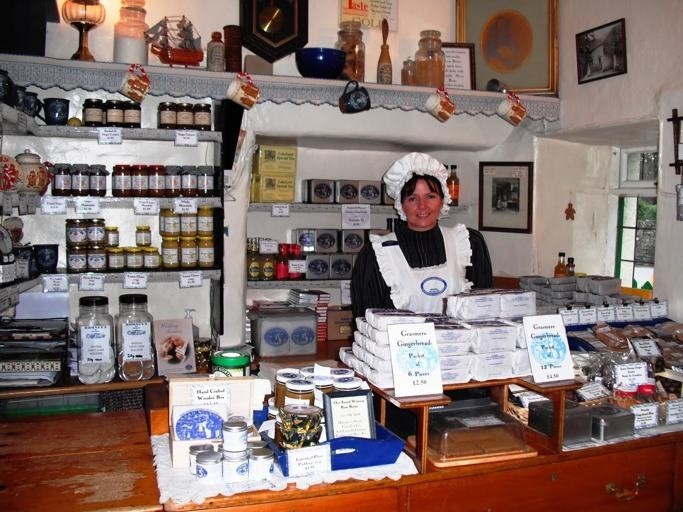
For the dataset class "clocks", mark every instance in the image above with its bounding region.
[235,2,312,64]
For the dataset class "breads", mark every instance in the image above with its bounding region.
[594,320,683,409]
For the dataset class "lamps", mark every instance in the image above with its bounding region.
[59,1,105,64]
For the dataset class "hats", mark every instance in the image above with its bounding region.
[383,151,452,222]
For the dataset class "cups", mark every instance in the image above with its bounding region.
[224,74,261,110]
[0,69,70,126]
[33,244,59,274]
[497,94,527,128]
[423,87,457,123]
[117,65,152,105]
[13,245,36,280]
[338,80,371,114]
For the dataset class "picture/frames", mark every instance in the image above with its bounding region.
[573,17,630,86]
[437,40,476,90]
[450,0,561,100]
[474,160,537,236]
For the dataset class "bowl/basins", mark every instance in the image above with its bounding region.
[294,47,345,80]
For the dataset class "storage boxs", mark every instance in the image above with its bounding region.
[252,305,318,359]
[326,302,353,340]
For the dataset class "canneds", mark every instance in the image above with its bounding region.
[83,99,141,126]
[191,366,365,484]
[53,164,105,196]
[66,206,213,272]
[113,164,214,196]
[248,244,303,281]
[159,101,211,128]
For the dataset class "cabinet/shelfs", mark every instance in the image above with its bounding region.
[1,369,409,510]
[16,124,228,369]
[1,102,47,302]
[369,371,682,511]
[242,197,472,346]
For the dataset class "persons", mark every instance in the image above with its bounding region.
[346,152,499,320]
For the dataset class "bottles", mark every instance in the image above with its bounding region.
[334,20,365,82]
[443,164,448,178]
[414,28,446,89]
[276,241,303,281]
[223,24,242,73]
[115,294,155,382]
[245,236,277,281]
[205,30,226,72]
[566,257,576,277]
[554,251,567,277]
[446,165,459,207]
[377,45,393,85]
[75,294,116,384]
[401,59,415,86]
